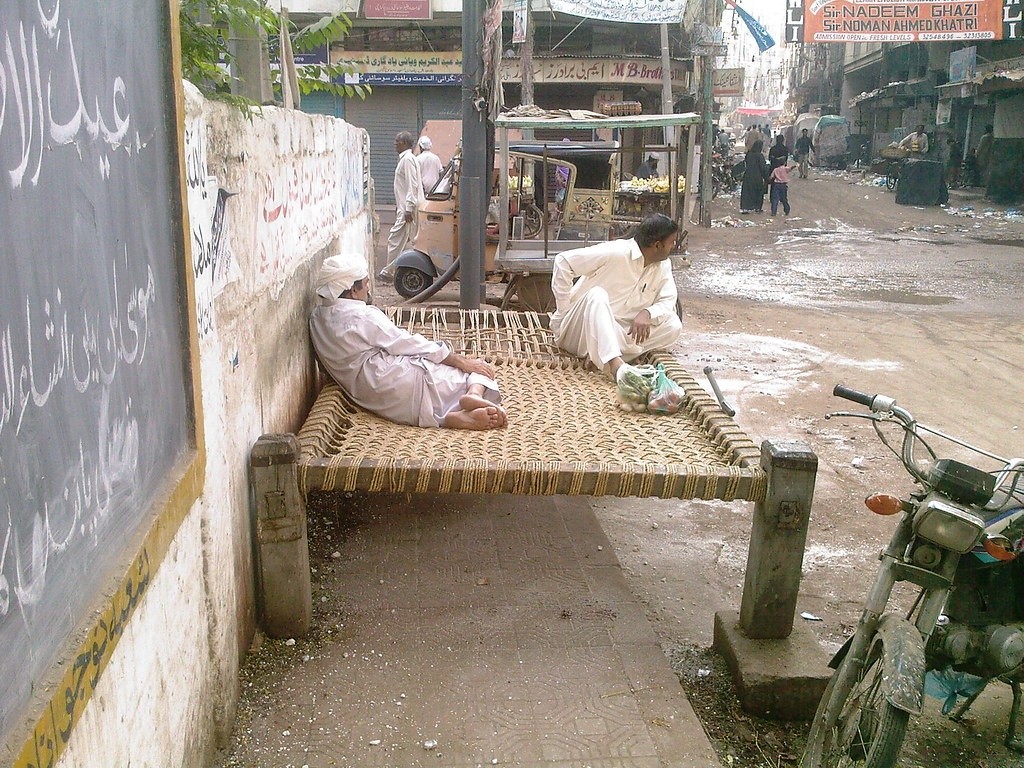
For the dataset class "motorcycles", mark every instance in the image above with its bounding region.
[802,384,1024,768]
[393,138,620,299]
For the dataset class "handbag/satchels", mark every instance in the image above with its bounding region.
[732,161,746,182]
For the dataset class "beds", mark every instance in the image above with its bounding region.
[246,299,817,639]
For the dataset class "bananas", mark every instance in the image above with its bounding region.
[630,175,686,194]
[508,175,533,188]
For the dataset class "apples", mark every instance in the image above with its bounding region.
[647,389,682,415]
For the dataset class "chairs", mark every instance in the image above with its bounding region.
[487,196,518,234]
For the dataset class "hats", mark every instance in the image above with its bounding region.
[417,136,432,150]
[650,151,659,160]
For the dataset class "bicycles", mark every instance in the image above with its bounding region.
[886,146,913,190]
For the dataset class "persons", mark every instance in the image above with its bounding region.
[718,124,815,216]
[416,136,444,195]
[899,125,928,159]
[976,124,993,187]
[946,138,961,190]
[549,214,683,382]
[309,251,507,431]
[635,152,660,180]
[376,131,425,283]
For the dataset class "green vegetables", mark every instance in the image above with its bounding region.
[615,371,657,404]
